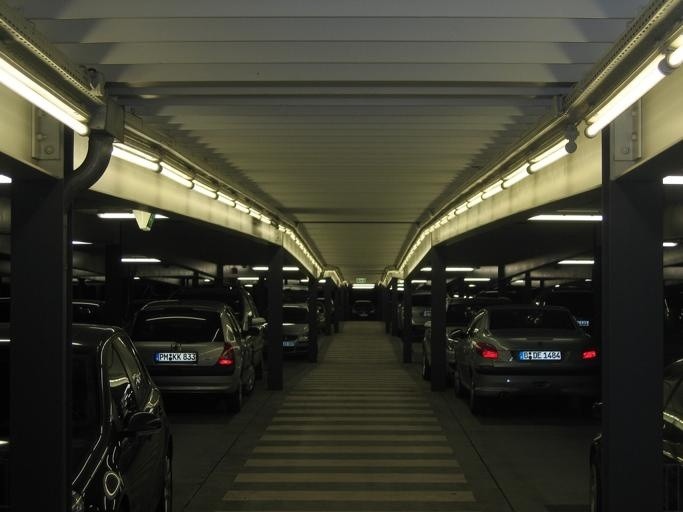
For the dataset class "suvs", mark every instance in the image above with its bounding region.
[422,297,514,386]
[167,286,269,379]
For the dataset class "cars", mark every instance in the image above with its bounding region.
[351,300,375,321]
[590,353,683,512]
[125,299,260,413]
[528,279,683,367]
[0,323,173,512]
[448,305,599,418]
[396,290,453,338]
[282,298,337,362]
[0,297,157,335]
